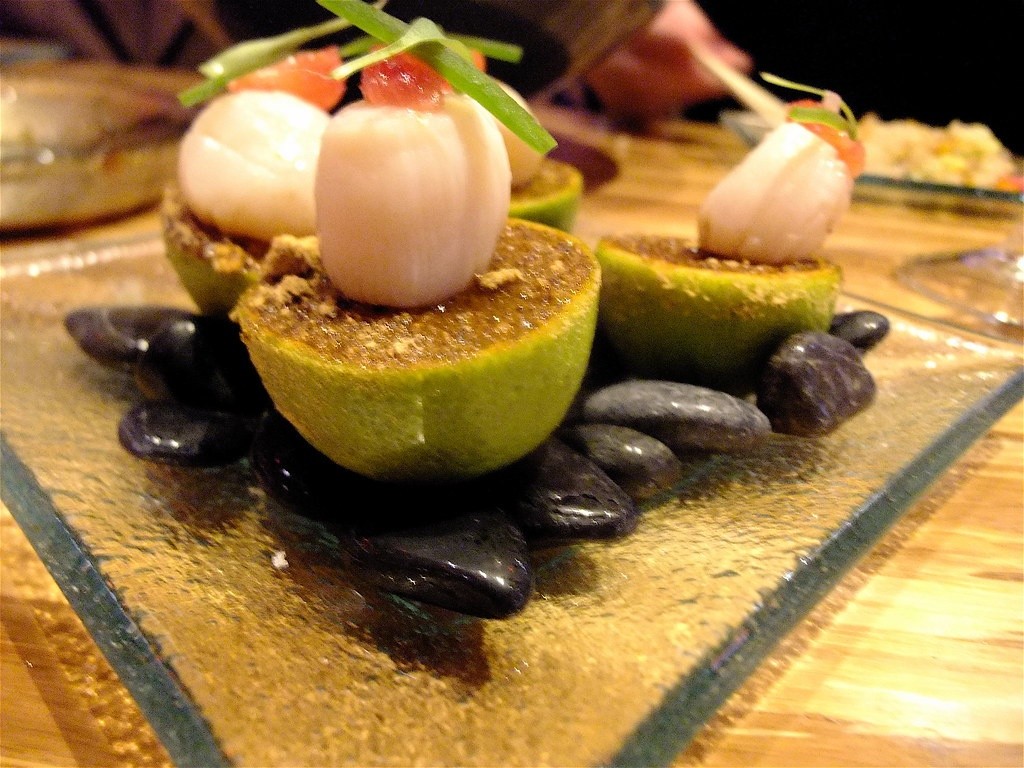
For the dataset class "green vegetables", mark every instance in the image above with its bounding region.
[178,0,860,152]
